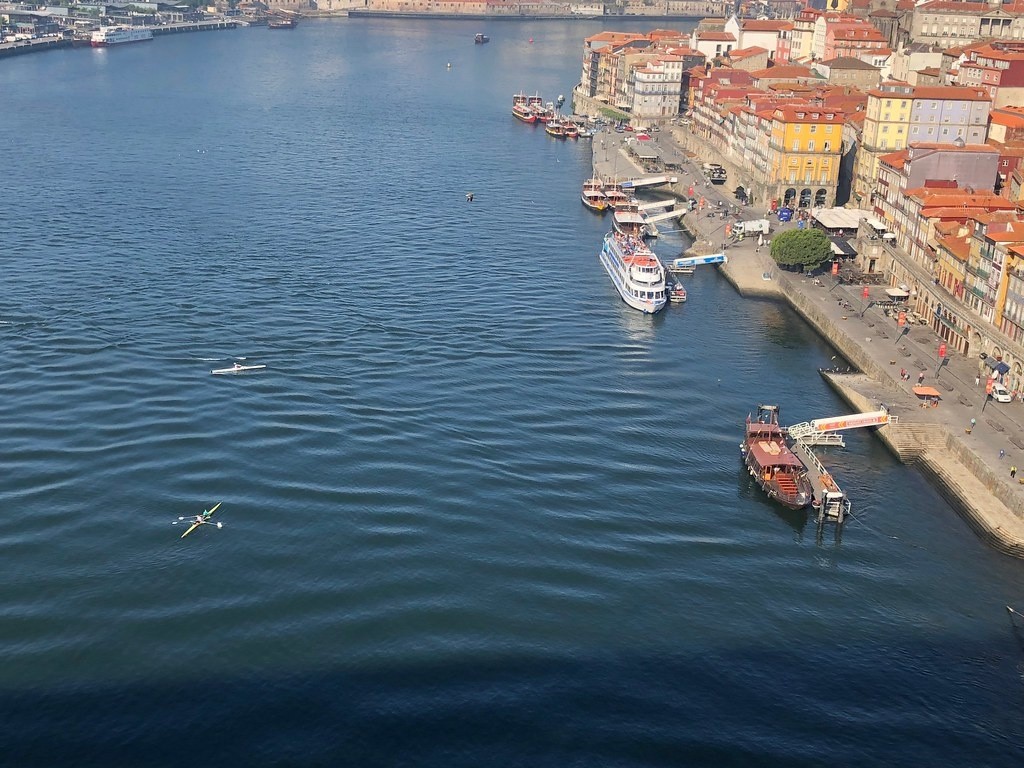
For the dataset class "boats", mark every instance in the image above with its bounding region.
[612,210,646,241]
[528,91,554,122]
[581,169,608,212]
[599,231,667,314]
[512,90,536,122]
[559,115,578,137]
[270,19,293,29]
[739,403,812,511]
[638,210,658,236]
[91,25,153,47]
[474,34,489,43]
[545,111,563,136]
[602,169,631,211]
[555,102,562,108]
[558,95,565,101]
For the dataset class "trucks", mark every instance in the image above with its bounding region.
[779,208,791,221]
[732,219,770,237]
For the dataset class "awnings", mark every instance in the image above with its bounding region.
[984,356,1011,374]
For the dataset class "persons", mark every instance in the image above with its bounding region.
[610,230,642,255]
[837,298,848,308]
[688,198,744,242]
[767,202,845,237]
[971,374,1024,478]
[900,367,938,407]
[601,129,658,146]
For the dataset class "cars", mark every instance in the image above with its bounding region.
[595,120,659,133]
[990,382,1011,403]
[0,27,74,44]
